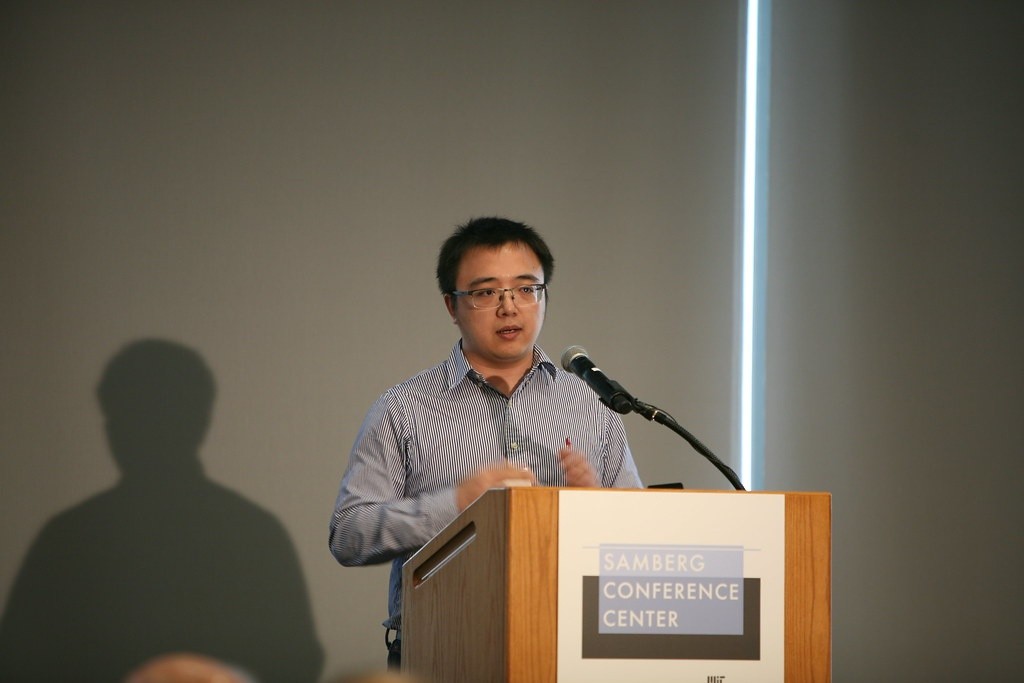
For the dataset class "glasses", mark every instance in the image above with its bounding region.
[450,277,549,313]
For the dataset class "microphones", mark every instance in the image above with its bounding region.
[560,345,632,415]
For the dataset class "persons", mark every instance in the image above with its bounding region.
[328,216,643,672]
